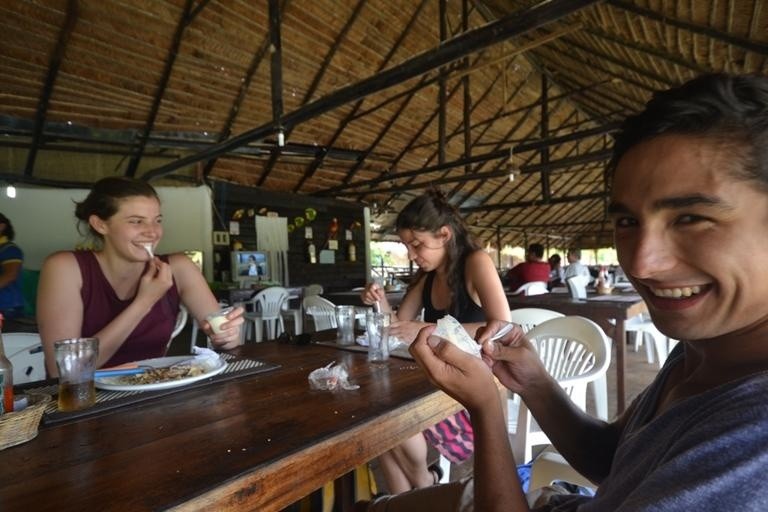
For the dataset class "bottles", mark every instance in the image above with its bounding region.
[0,314,13,415]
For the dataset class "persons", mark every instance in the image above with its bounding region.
[364,75,768,511]
[542,255,561,284]
[559,249,592,286]
[0,210,25,336]
[36,178,244,381]
[360,190,511,494]
[505,244,548,293]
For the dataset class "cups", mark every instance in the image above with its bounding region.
[203,306,235,336]
[334,305,355,345]
[53,337,101,410]
[365,313,391,361]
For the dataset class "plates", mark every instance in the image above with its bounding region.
[94,355,228,391]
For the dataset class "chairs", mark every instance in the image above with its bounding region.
[302,294,338,332]
[439,315,611,484]
[190,303,229,354]
[565,275,587,299]
[304,284,323,297]
[638,310,680,368]
[244,286,290,342]
[506,281,548,297]
[280,286,304,336]
[162,304,188,357]
[506,308,613,434]
[606,313,644,352]
[0,333,47,386]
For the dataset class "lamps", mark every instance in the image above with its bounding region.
[7,182,16,198]
[506,161,517,182]
[278,127,285,147]
